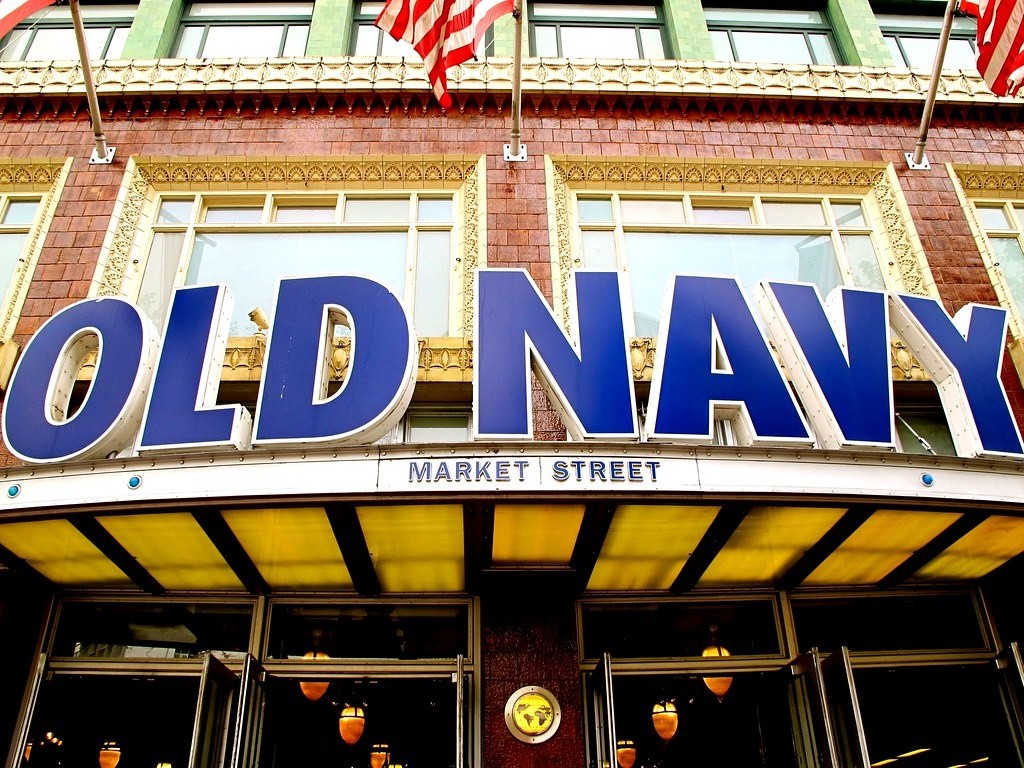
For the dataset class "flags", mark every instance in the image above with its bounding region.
[375,0,516,109]
[958,0,1024,99]
[0,0,55,38]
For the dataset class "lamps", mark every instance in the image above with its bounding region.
[701,627,734,702]
[248,304,269,337]
[617,733,638,768]
[98,737,121,768]
[300,628,331,701]
[648,687,682,745]
[370,740,390,768]
[339,695,366,745]
